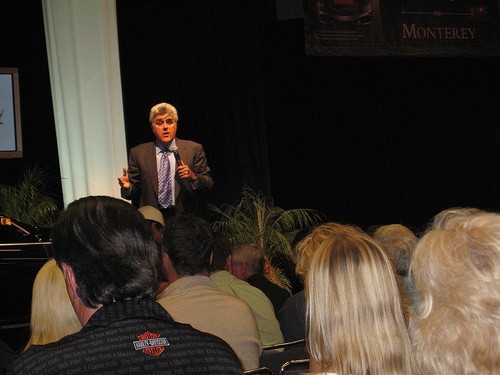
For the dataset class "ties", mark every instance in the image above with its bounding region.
[157,147,172,209]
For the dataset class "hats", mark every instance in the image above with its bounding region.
[137,205,165,228]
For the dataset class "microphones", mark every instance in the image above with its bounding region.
[172,146,182,167]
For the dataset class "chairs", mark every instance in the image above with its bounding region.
[241,338,310,375]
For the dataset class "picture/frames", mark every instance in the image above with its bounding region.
[0,67,23,158]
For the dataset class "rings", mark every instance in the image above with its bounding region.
[185,171,188,174]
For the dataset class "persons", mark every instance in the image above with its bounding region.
[137,205,164,245]
[279,222,395,343]
[153,214,263,372]
[25,257,83,356]
[6,196,245,375]
[305,233,418,375]
[118,102,212,222]
[209,233,285,353]
[230,243,292,318]
[372,222,421,308]
[409,207,500,375]
